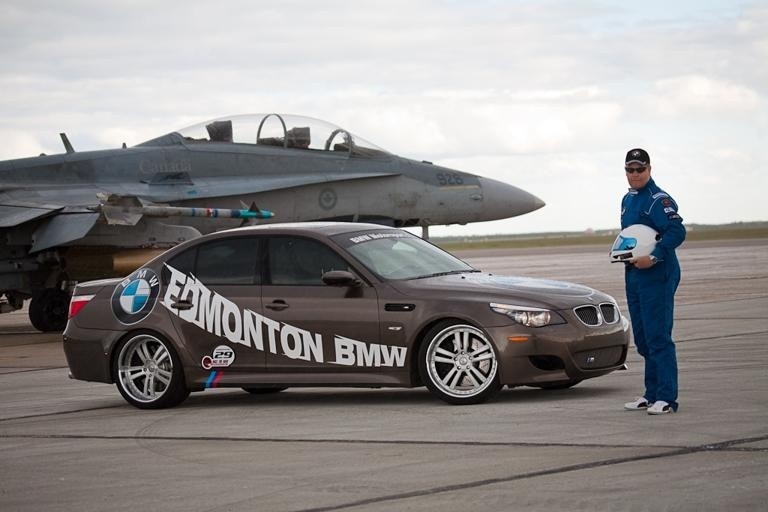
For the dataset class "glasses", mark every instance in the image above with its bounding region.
[625,167,647,173]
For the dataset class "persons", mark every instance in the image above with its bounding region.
[606,148,687,417]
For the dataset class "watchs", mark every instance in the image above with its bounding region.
[648,254,659,267]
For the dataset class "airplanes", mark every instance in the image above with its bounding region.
[0,113,545,333]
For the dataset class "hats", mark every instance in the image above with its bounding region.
[625,148,650,165]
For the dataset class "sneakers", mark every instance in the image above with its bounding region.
[647,400,674,415]
[624,396,654,410]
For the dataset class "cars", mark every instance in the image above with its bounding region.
[62,222,631,409]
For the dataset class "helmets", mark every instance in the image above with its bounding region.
[610,224,665,264]
